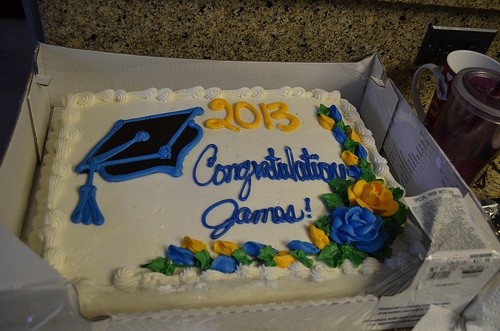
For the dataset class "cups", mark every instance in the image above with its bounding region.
[411,50,500,133]
[432,67,500,187]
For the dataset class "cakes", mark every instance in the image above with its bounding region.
[28,86,427,321]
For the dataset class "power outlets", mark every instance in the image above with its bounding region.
[413,23,497,67]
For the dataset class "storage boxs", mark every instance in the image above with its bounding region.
[0,42,500,331]
